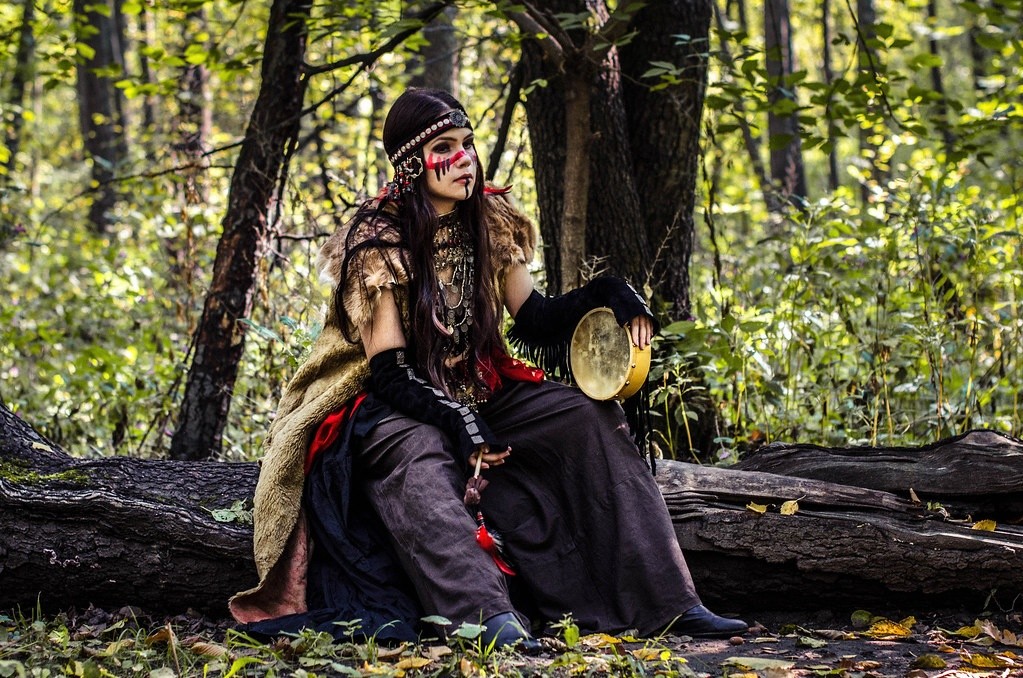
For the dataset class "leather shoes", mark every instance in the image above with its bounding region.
[457,612,543,656]
[644,605,747,638]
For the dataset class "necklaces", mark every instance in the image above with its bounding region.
[425,213,474,334]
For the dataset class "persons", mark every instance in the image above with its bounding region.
[227,87,748,657]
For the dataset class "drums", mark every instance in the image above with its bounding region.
[568,306,652,403]
